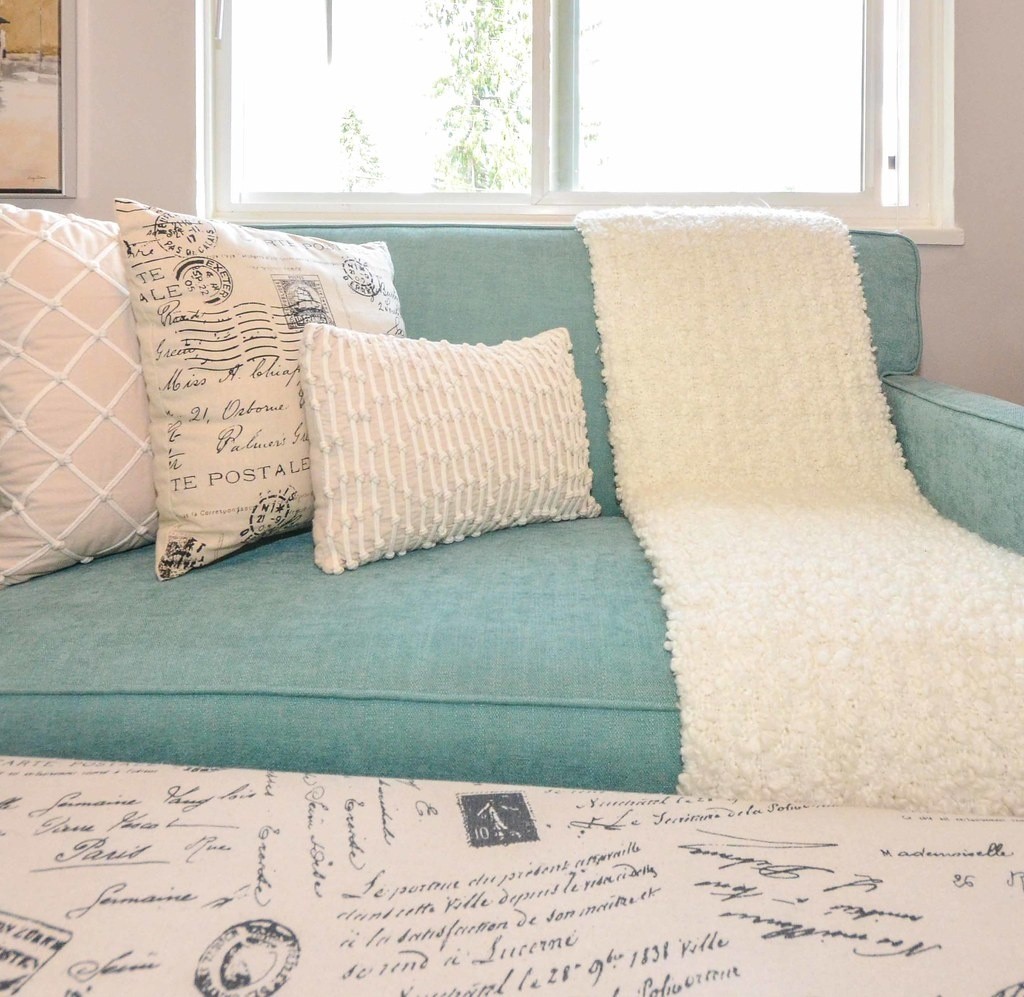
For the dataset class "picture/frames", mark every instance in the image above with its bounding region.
[0,0,78,200]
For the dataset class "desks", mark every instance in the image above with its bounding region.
[0,755,1024,997]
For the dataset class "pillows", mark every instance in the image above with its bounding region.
[301,322,604,575]
[113,195,407,583]
[0,205,159,592]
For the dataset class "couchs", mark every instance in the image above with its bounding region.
[0,202,1024,818]
